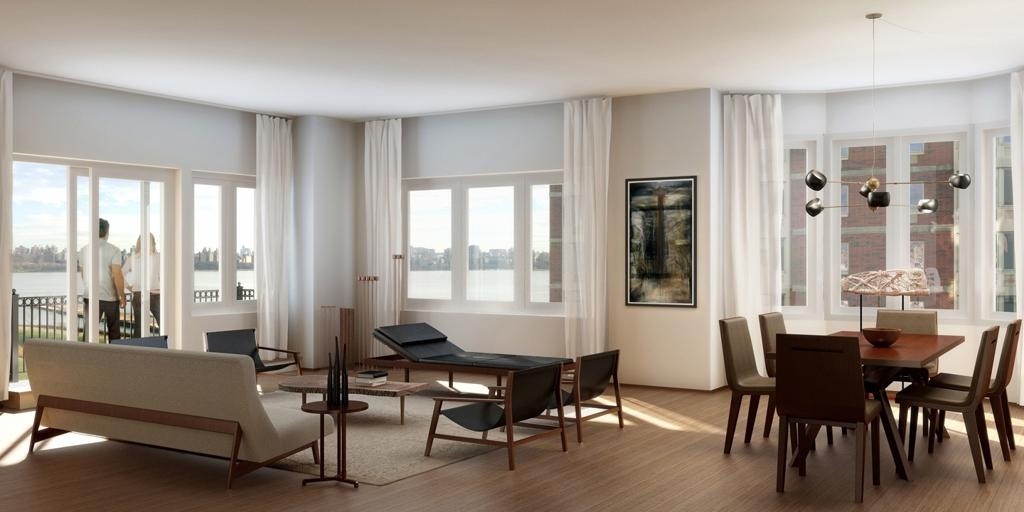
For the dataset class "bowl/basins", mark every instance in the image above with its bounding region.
[862,328,902,347]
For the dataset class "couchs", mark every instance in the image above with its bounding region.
[21,339,336,490]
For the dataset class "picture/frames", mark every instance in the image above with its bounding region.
[625,176,696,308]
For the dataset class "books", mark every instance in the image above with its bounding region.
[355,371,389,388]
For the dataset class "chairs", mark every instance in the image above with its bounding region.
[547,349,623,443]
[719,316,798,454]
[353,322,579,383]
[759,311,787,438]
[894,325,1001,483]
[110,335,168,347]
[929,320,1022,462]
[203,329,302,376]
[424,362,567,471]
[775,333,880,503]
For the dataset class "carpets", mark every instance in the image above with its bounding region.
[266,380,619,488]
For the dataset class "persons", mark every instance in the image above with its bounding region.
[121,232,160,338]
[80,218,126,344]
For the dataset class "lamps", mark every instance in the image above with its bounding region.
[804,13,971,217]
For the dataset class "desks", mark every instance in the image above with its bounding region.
[301,399,368,488]
